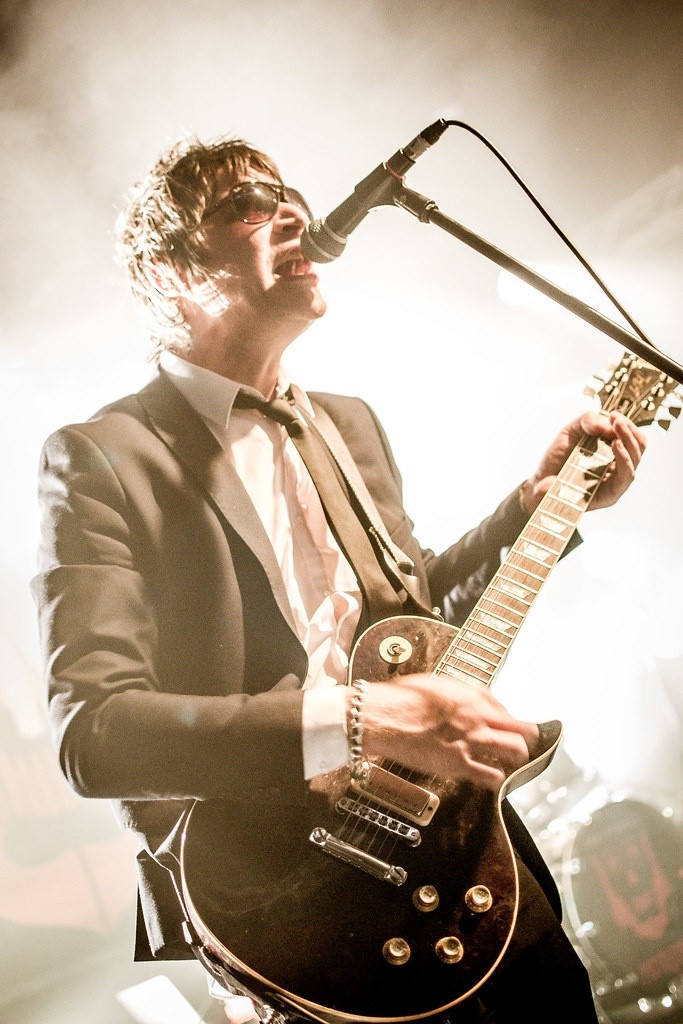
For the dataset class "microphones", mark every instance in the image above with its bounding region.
[300,119,449,264]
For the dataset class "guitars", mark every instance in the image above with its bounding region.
[178,352,683,1024]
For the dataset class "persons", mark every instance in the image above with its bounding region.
[32,137,649,1022]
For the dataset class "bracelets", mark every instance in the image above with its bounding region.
[337,677,376,781]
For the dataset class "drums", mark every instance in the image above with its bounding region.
[562,792,683,1005]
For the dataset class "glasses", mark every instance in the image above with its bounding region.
[167,183,313,243]
